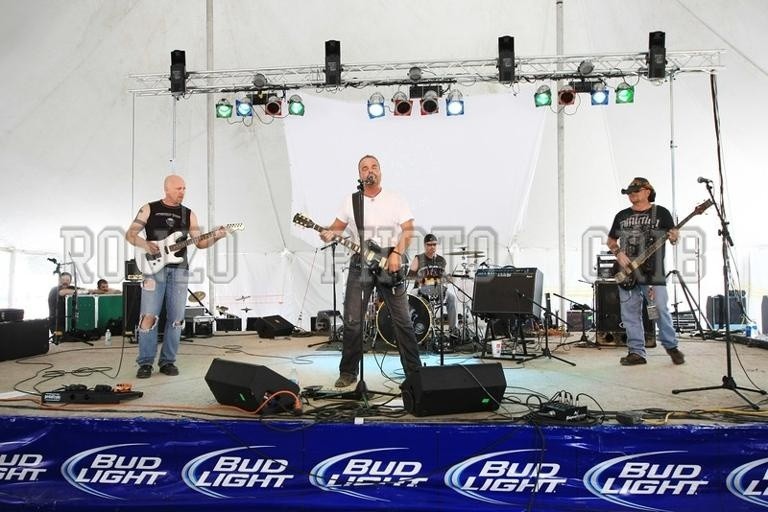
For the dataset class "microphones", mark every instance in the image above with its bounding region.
[47,258,57,264]
[697,177,711,183]
[514,287,525,298]
[367,173,376,185]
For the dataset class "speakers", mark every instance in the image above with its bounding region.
[217,319,241,331]
[247,318,260,331]
[122,282,166,335]
[401,362,506,417]
[471,269,544,319]
[0,320,49,363]
[596,282,656,348]
[205,358,300,415]
[256,315,294,338]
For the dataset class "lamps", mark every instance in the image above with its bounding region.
[534,60,635,110]
[214,73,306,124]
[365,67,464,119]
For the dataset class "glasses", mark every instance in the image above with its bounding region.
[427,243,437,247]
[627,187,648,194]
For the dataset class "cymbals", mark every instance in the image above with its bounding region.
[189,292,206,303]
[237,296,250,301]
[241,308,250,311]
[446,251,484,255]
[467,254,485,258]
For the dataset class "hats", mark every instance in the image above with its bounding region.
[621,178,653,193]
[424,234,437,243]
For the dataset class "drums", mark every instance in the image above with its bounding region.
[417,267,445,287]
[376,294,433,347]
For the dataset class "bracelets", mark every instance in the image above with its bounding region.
[390,248,404,258]
[669,238,680,245]
[612,248,622,257]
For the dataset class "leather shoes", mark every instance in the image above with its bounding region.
[666,347,685,364]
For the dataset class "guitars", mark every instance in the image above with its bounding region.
[134,223,245,275]
[611,199,713,289]
[292,213,409,286]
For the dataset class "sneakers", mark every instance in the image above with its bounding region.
[335,372,357,387]
[450,328,459,337]
[137,366,151,378]
[414,322,425,334]
[160,363,179,375]
[620,353,646,365]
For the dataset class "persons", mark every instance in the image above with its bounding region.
[125,175,228,379]
[607,178,685,365]
[408,235,458,332]
[91,280,121,295]
[48,272,92,334]
[319,154,421,388]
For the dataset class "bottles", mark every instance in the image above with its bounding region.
[104,329,112,346]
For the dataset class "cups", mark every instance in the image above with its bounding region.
[490,339,502,358]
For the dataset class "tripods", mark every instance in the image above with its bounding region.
[313,192,401,409]
[557,309,602,350]
[672,236,768,410]
[516,314,576,366]
[65,267,94,346]
[49,275,65,343]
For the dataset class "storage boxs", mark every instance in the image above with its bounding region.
[565,308,594,331]
[184,306,204,338]
[65,294,123,331]
[193,316,217,338]
